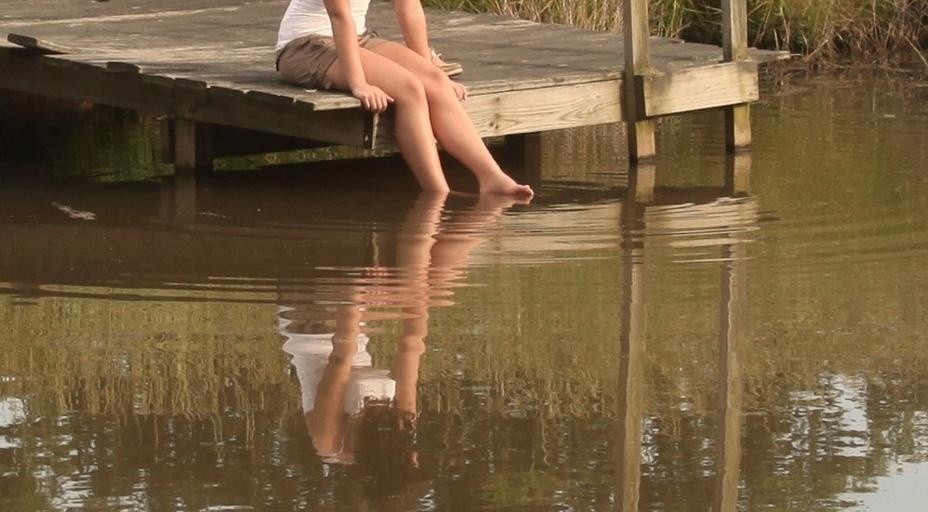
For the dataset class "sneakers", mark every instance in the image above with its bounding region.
[431,47,463,77]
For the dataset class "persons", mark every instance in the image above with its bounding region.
[275,0,535,196]
[279,195,533,512]
